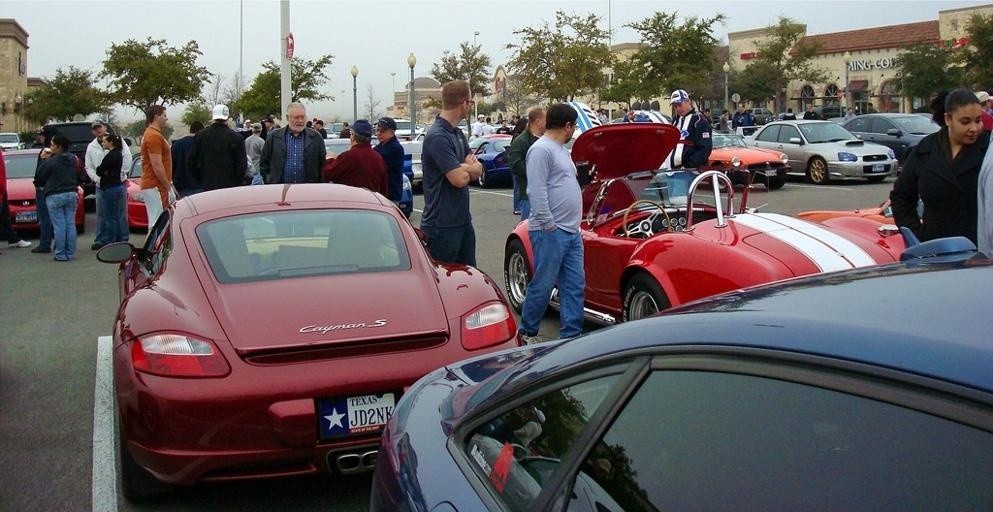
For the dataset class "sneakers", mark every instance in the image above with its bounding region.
[91,243,100,249]
[9,240,32,247]
[513,210,522,214]
[515,329,543,347]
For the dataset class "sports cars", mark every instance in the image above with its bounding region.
[502,120,907,327]
[94,181,521,502]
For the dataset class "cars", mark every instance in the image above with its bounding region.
[0,132,25,152]
[733,124,763,144]
[556,100,603,187]
[468,122,515,151]
[700,131,792,189]
[369,228,993,511]
[319,118,424,191]
[126,153,148,231]
[736,118,900,181]
[800,196,925,226]
[467,139,515,189]
[609,109,673,124]
[3,148,87,235]
[843,113,944,159]
[712,109,731,123]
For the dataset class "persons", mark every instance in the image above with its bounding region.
[803,104,820,120]
[510,108,546,222]
[511,407,546,450]
[471,111,528,145]
[783,108,796,120]
[667,90,713,208]
[597,107,635,123]
[747,110,756,126]
[0,145,32,248]
[843,108,856,121]
[731,101,752,134]
[888,89,993,259]
[588,457,613,483]
[719,110,730,134]
[700,109,712,126]
[421,81,483,269]
[515,104,587,347]
[868,103,877,113]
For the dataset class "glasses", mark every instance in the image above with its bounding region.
[460,99,475,108]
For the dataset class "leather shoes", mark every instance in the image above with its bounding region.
[31,248,51,253]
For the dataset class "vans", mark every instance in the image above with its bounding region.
[457,119,469,135]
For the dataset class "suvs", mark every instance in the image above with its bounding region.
[31,122,134,197]
[813,104,845,120]
[744,107,775,124]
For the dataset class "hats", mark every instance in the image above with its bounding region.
[36,127,45,134]
[91,119,104,129]
[315,120,323,125]
[265,118,273,123]
[974,91,993,102]
[373,117,396,130]
[347,119,372,136]
[253,122,261,129]
[669,89,690,106]
[212,104,229,120]
[478,114,484,118]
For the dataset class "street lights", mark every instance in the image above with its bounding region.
[842,51,853,115]
[406,53,418,141]
[473,29,483,121]
[351,66,359,126]
[721,61,730,112]
